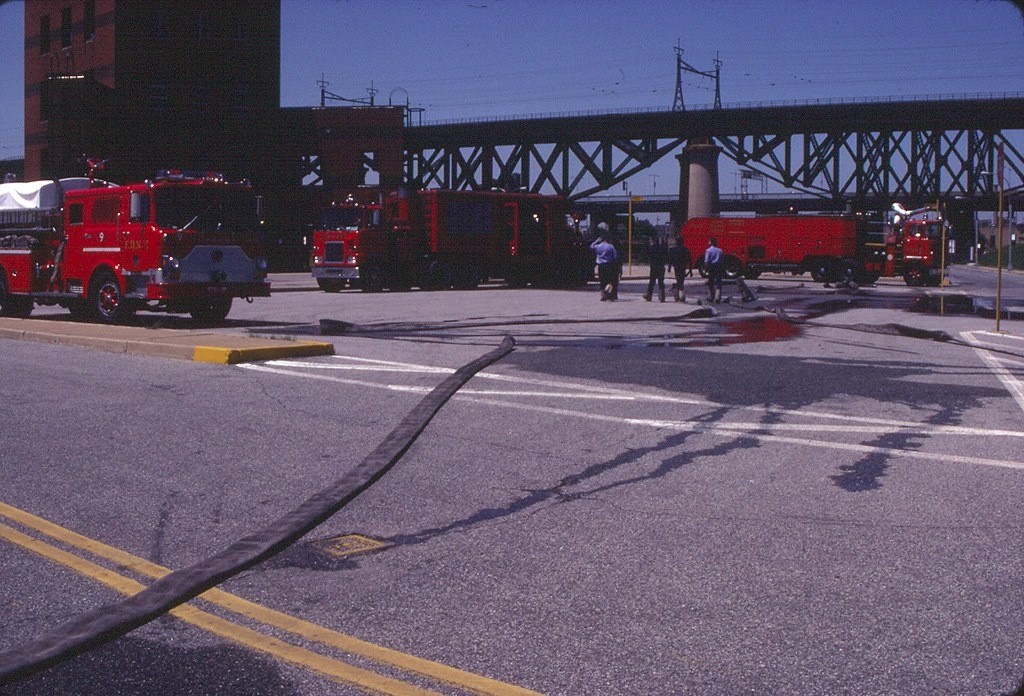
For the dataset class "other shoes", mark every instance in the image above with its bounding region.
[659,298,665,302]
[643,295,651,301]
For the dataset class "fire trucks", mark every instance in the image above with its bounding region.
[307,176,597,294]
[675,202,956,288]
[0,152,273,326]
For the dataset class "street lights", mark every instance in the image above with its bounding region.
[955,195,979,267]
[981,171,1013,270]
[649,174,661,195]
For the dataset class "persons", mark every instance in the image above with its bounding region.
[641,235,665,302]
[589,235,619,302]
[667,235,693,302]
[702,237,723,304]
